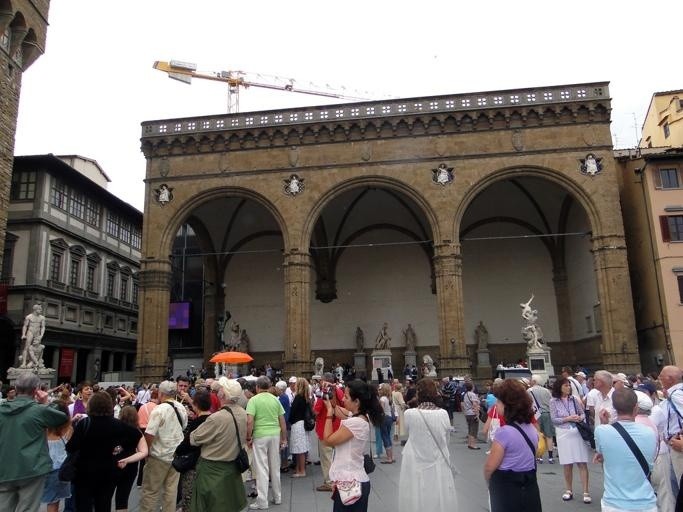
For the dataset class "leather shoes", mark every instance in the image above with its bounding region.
[468,445,481,449]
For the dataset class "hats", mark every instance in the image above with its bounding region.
[575,371,587,378]
[519,377,531,387]
[158,380,177,396]
[613,373,629,384]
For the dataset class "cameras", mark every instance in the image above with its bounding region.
[316,390,334,401]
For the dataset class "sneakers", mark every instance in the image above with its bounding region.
[548,458,555,465]
[373,454,396,464]
[536,457,544,464]
[562,489,573,500]
[316,484,333,492]
[271,497,281,505]
[583,492,591,503]
[290,473,307,478]
[249,501,269,510]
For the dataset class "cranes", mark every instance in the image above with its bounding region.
[151,59,399,114]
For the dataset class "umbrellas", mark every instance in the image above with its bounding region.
[209,351,253,363]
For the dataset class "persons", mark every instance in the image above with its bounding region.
[355,326,365,352]
[402,323,416,351]
[158,185,170,205]
[375,322,391,349]
[20,304,46,368]
[436,164,449,186]
[240,329,249,351]
[585,155,598,176]
[26,336,47,369]
[475,321,489,349]
[289,175,299,195]
[220,310,239,351]
[520,293,543,349]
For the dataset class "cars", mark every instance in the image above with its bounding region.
[441,375,477,398]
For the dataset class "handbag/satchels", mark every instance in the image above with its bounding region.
[303,403,315,431]
[236,447,249,473]
[576,421,592,441]
[336,479,363,507]
[58,452,82,481]
